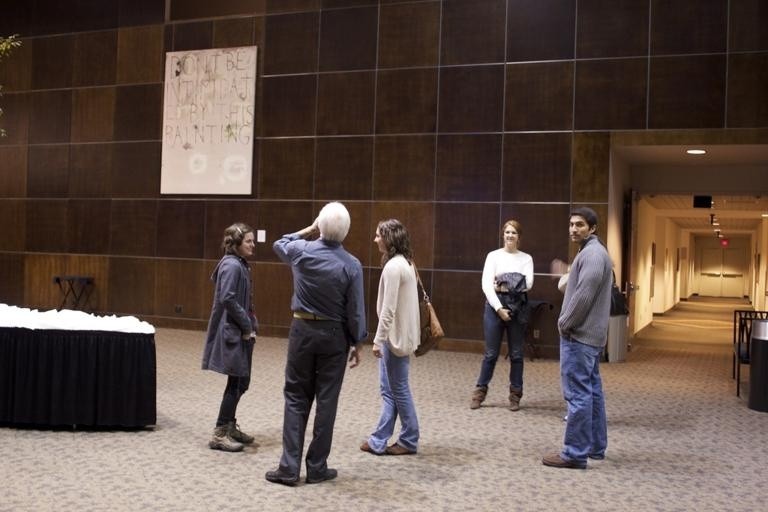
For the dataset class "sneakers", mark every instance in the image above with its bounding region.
[385,443,416,455]
[360,441,383,454]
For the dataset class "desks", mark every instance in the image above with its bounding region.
[748,320,768,411]
[55,275,95,315]
[504,301,553,362]
[0,302,157,432]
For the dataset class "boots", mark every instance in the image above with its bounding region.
[510,385,522,411]
[470,385,487,409]
[210,417,254,452]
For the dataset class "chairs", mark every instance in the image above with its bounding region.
[731,311,768,397]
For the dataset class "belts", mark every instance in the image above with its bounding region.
[293,311,330,321]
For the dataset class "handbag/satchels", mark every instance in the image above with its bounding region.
[413,296,444,357]
[610,270,629,317]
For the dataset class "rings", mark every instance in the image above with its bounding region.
[375,354,378,356]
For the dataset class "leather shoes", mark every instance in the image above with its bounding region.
[306,469,337,483]
[266,471,299,483]
[543,454,586,469]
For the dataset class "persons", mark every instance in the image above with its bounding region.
[542,207,613,468]
[265,199,369,481]
[200,225,259,451]
[360,219,423,456]
[470,221,534,409]
